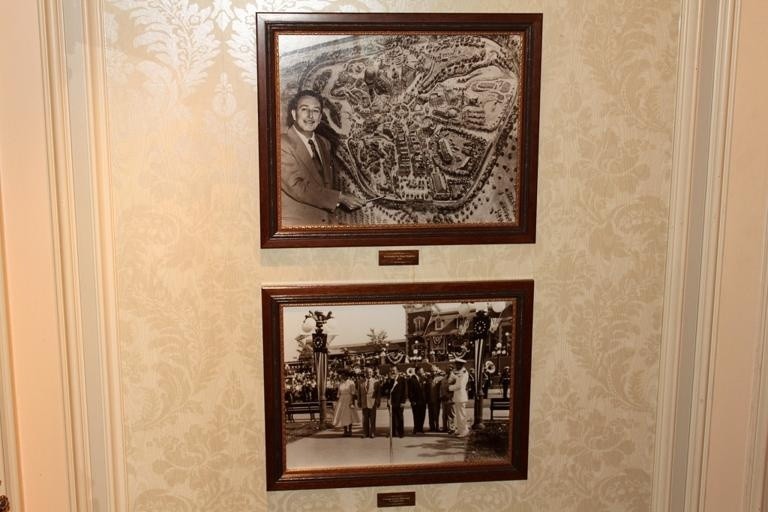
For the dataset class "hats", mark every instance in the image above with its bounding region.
[453,357,467,364]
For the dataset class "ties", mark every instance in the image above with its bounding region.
[366,380,369,391]
[306,138,323,176]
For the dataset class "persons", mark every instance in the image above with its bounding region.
[278,88,371,227]
[279,340,511,442]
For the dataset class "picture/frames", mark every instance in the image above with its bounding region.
[256,13,543,249]
[260,279,535,492]
